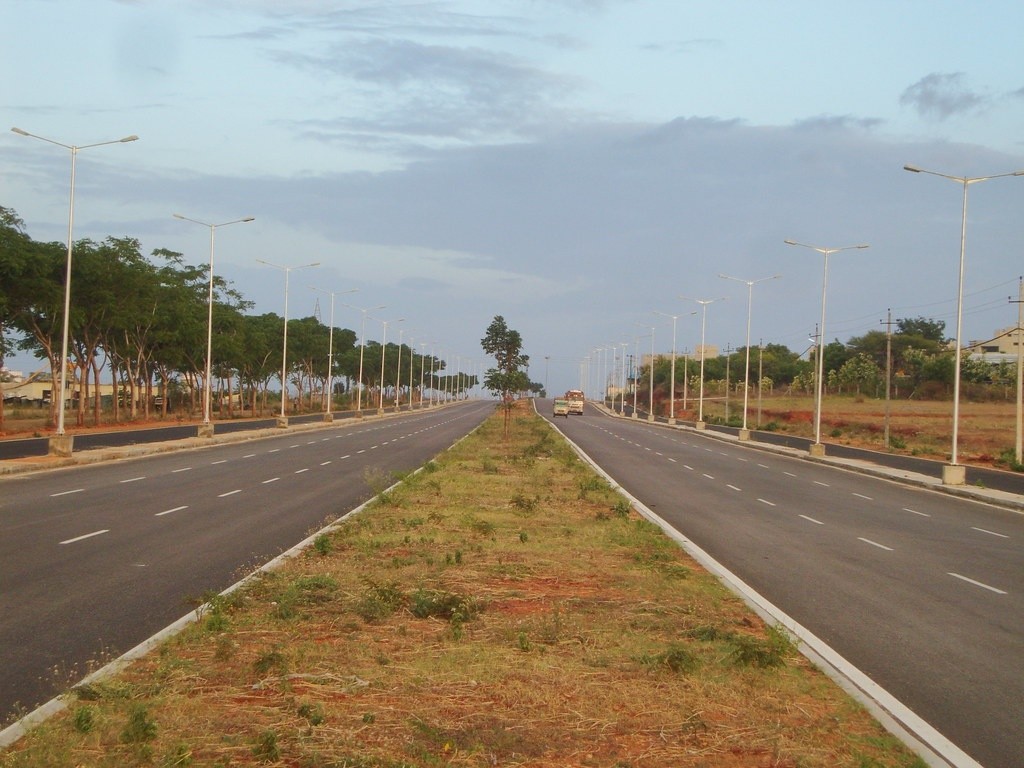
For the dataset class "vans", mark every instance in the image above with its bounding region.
[154,398,174,413]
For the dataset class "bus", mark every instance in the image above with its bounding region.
[565,389,584,414]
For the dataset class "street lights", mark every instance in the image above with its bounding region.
[579,306,698,425]
[171,213,256,438]
[675,293,732,431]
[903,164,1024,485]
[11,126,140,456]
[717,272,783,441]
[256,259,322,429]
[783,239,870,456]
[309,285,478,422]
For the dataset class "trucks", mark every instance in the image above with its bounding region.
[552,397,569,419]
